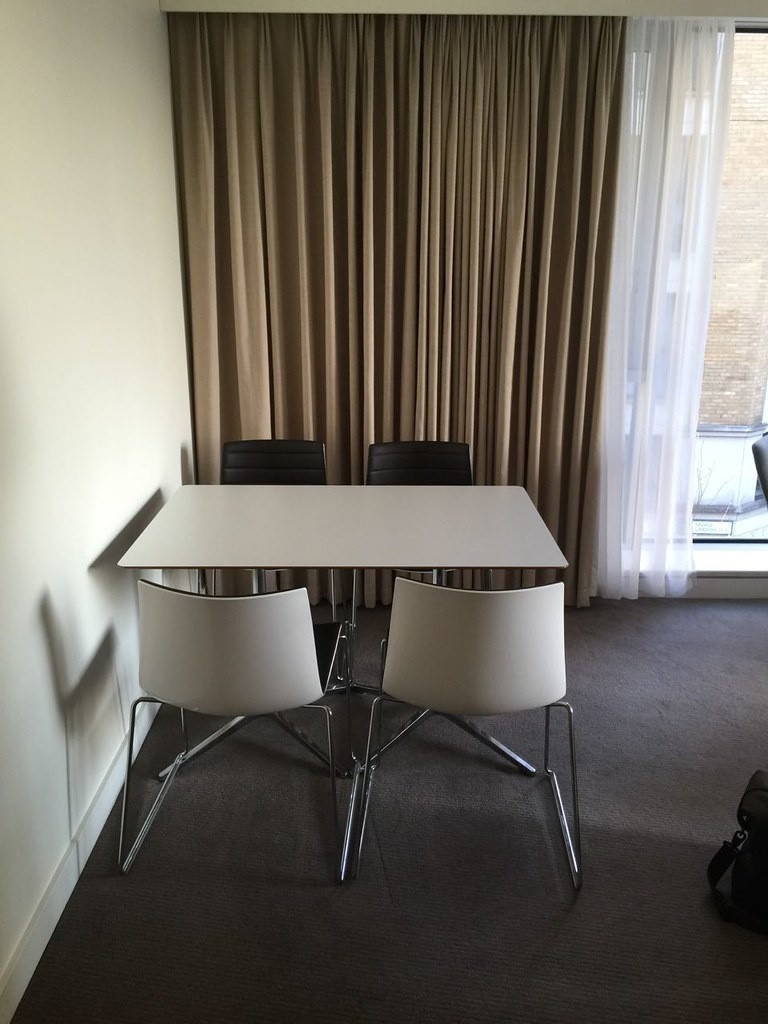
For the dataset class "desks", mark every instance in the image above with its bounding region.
[117,484,570,782]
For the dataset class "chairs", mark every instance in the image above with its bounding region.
[114,440,584,891]
